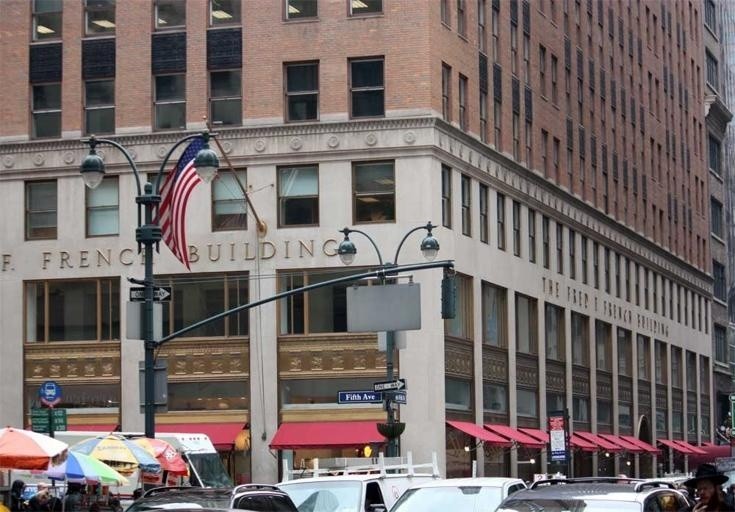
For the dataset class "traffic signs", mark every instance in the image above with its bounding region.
[31,407,67,433]
[337,391,383,404]
[393,393,406,405]
[128,285,174,302]
[374,379,406,392]
[39,381,62,406]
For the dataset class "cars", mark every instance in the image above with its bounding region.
[386,477,529,512]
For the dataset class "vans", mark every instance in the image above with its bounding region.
[11,431,233,508]
[274,473,444,512]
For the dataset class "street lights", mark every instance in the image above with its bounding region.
[336,220,438,458]
[79,133,220,439]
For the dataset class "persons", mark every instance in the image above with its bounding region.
[0,481,122,511]
[681,464,734,512]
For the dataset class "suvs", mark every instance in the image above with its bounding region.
[123,483,298,511]
[495,475,688,512]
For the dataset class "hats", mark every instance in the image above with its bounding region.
[684,464,729,489]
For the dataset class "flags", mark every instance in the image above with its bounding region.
[147,130,214,269]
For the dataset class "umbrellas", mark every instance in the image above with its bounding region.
[0,424,191,487]
